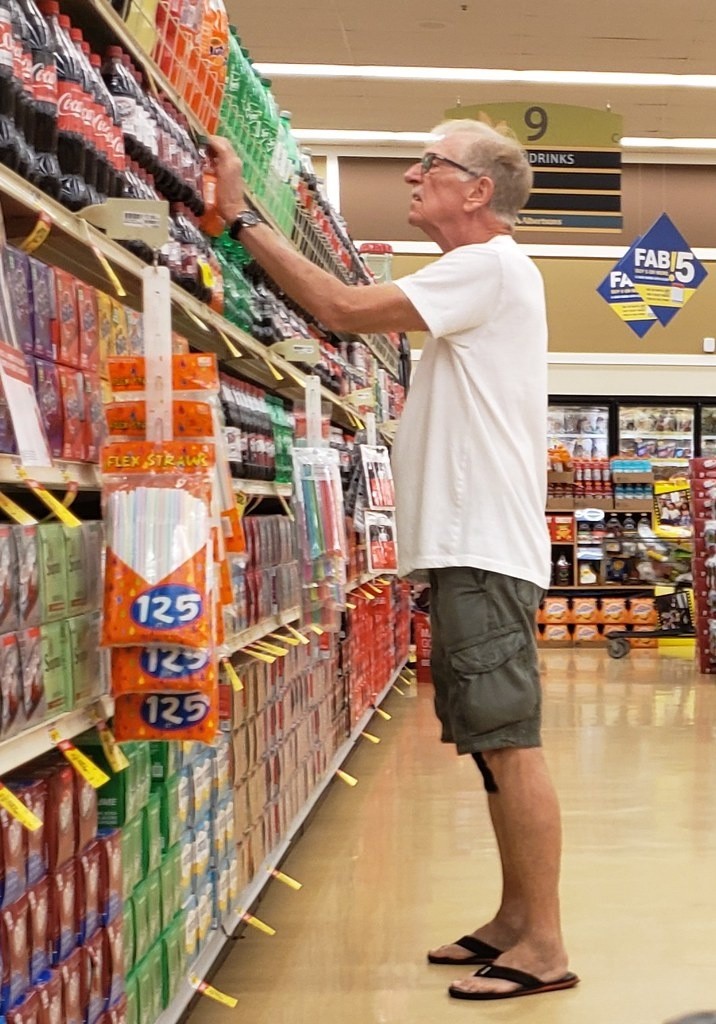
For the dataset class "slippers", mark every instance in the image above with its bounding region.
[428,935,505,965]
[448,963,578,1001]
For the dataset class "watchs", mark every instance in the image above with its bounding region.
[228,210,261,240]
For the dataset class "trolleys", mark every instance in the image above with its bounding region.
[603,626,695,659]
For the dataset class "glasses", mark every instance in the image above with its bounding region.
[422,152,482,179]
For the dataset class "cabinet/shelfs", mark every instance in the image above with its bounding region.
[548,455,716,647]
[0,0,411,1024]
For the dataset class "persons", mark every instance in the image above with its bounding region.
[208,119,580,1001]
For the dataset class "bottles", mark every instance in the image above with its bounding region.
[546,446,654,499]
[606,514,622,531]
[555,551,571,587]
[0,0,372,395]
[638,512,651,532]
[624,513,636,530]
[215,371,295,482]
